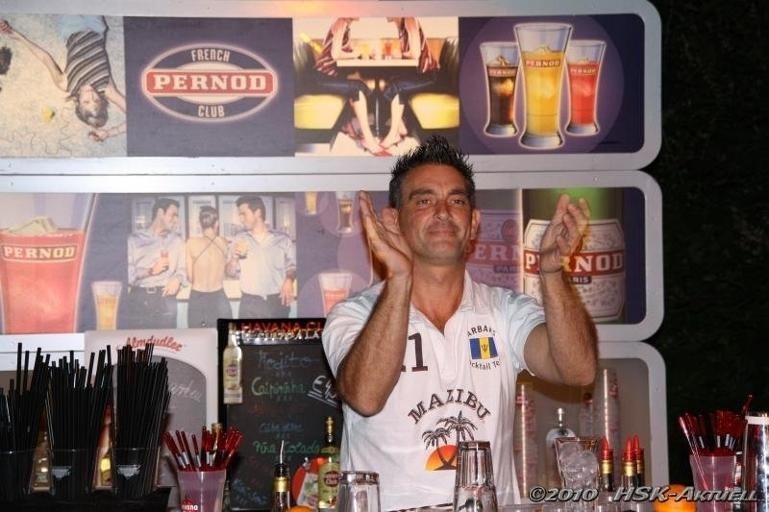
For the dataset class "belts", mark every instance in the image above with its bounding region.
[242,294,281,300]
[132,288,166,295]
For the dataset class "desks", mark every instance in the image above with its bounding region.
[0,487,172,512]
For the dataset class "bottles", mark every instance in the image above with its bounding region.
[222,322,243,405]
[597,435,653,512]
[316,416,343,512]
[543,406,578,507]
[271,460,291,512]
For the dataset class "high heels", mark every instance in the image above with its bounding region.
[363,145,391,157]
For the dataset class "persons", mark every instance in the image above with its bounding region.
[10,14,127,142]
[226,196,296,320]
[0,20,12,75]
[125,198,186,329]
[320,132,600,511]
[380,17,438,148]
[187,204,235,328]
[307,17,384,154]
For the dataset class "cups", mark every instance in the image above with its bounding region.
[109,444,162,502]
[512,22,573,150]
[333,190,356,236]
[89,281,123,332]
[478,40,523,139]
[740,414,769,511]
[452,439,498,512]
[564,39,607,138]
[334,470,382,512]
[0,191,96,336]
[512,379,541,500]
[553,434,599,511]
[577,367,623,500]
[686,452,737,512]
[42,444,104,502]
[0,442,38,509]
[317,273,352,318]
[176,466,226,512]
